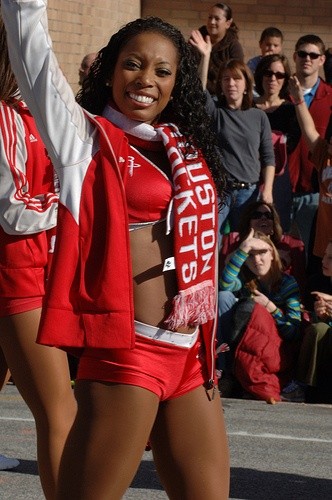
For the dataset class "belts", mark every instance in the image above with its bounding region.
[231,181,258,189]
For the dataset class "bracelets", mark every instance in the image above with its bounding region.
[264,300,270,308]
[295,100,304,105]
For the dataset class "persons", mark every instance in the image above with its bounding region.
[0,0,230,500]
[0,18,80,500]
[227,200,305,273]
[289,75,332,271]
[187,30,276,233]
[221,227,302,397]
[247,27,284,98]
[279,241,332,406]
[189,3,244,104]
[248,54,301,232]
[79,53,99,85]
[287,34,332,196]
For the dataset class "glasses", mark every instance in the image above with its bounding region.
[249,210,274,220]
[295,51,322,59]
[264,70,287,79]
